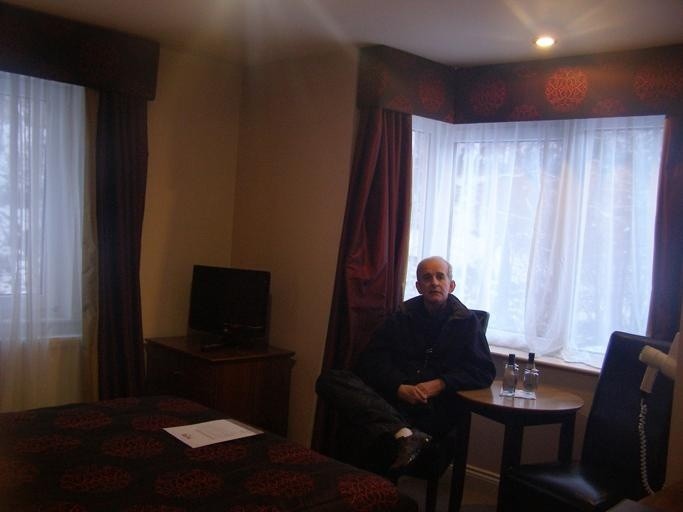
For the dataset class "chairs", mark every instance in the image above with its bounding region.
[509,329,674,511]
[388,308,490,512]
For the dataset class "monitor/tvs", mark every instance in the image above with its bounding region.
[187,264,271,352]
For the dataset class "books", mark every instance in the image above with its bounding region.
[161,417,266,448]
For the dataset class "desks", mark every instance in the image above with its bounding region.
[143,335,295,436]
[448,382,585,512]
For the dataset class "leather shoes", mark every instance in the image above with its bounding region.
[389,427,433,472]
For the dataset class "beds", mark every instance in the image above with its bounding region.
[1,391,407,512]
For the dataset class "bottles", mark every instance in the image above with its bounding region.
[502,354,520,397]
[521,353,541,397]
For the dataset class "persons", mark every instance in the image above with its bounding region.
[314,257,497,485]
